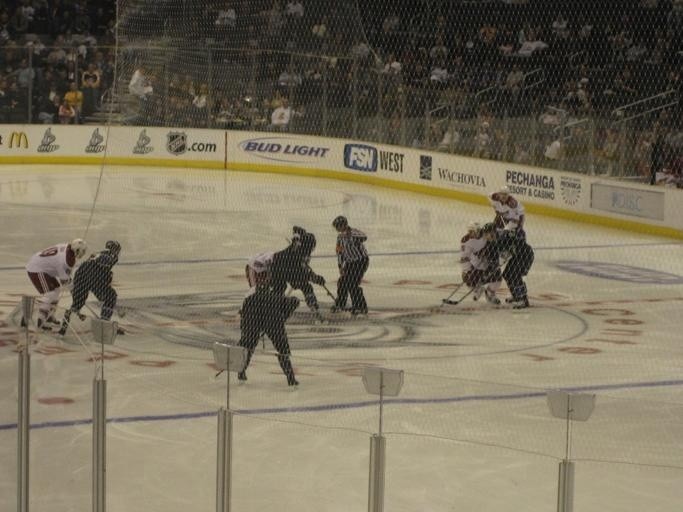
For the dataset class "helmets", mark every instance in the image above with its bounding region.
[467,222,480,237]
[71,239,85,256]
[498,186,509,195]
[482,223,497,235]
[105,240,121,254]
[256,284,269,293]
[332,215,347,227]
[301,233,315,250]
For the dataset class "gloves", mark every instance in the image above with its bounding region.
[313,275,324,286]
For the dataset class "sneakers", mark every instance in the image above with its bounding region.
[514,298,529,309]
[485,290,501,304]
[474,286,484,300]
[506,297,519,303]
[328,306,342,312]
[39,314,65,335]
[348,309,367,315]
[288,380,298,385]
[237,371,247,380]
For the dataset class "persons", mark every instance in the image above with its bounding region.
[0,0,683,190]
[234,282,302,387]
[458,189,536,313]
[23,237,88,333]
[61,238,122,328]
[242,214,369,314]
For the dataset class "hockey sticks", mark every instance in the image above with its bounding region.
[426,268,473,309]
[442,264,500,305]
[308,263,354,311]
